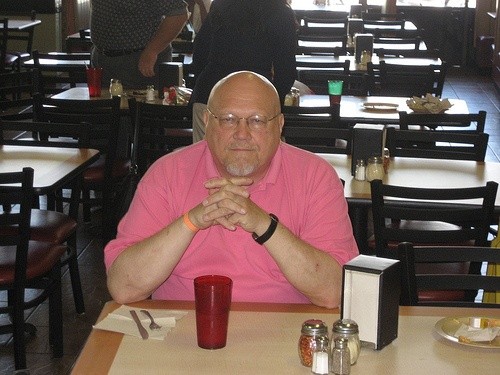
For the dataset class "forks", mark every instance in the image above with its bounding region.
[140,309,162,329]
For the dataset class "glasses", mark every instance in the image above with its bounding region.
[206,106,283,130]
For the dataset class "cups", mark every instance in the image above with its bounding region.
[86,67,104,97]
[328,80,344,107]
[194,275,233,350]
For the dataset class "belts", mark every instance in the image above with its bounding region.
[93,43,145,59]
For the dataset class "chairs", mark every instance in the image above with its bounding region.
[0,10,498,375]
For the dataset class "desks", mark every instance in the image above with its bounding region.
[69,299,499,375]
[294,37,427,52]
[297,17,418,30]
[297,95,470,128]
[0,146,100,312]
[314,152,500,255]
[48,86,191,235]
[293,55,439,94]
[23,50,192,86]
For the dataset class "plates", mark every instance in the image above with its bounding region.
[434,314,500,350]
[407,94,454,114]
[363,102,399,110]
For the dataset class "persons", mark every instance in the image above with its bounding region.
[86,0,300,146]
[104,70,360,309]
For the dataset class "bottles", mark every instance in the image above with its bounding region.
[347,32,359,47]
[299,319,332,368]
[330,336,351,375]
[355,159,367,180]
[285,88,300,108]
[360,50,371,64]
[311,336,331,375]
[366,156,384,182]
[330,319,360,366]
[108,79,124,97]
[145,84,155,104]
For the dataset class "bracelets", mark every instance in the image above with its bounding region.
[184,211,200,232]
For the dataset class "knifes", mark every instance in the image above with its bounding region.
[129,309,149,341]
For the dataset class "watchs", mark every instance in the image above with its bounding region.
[252,214,279,246]
